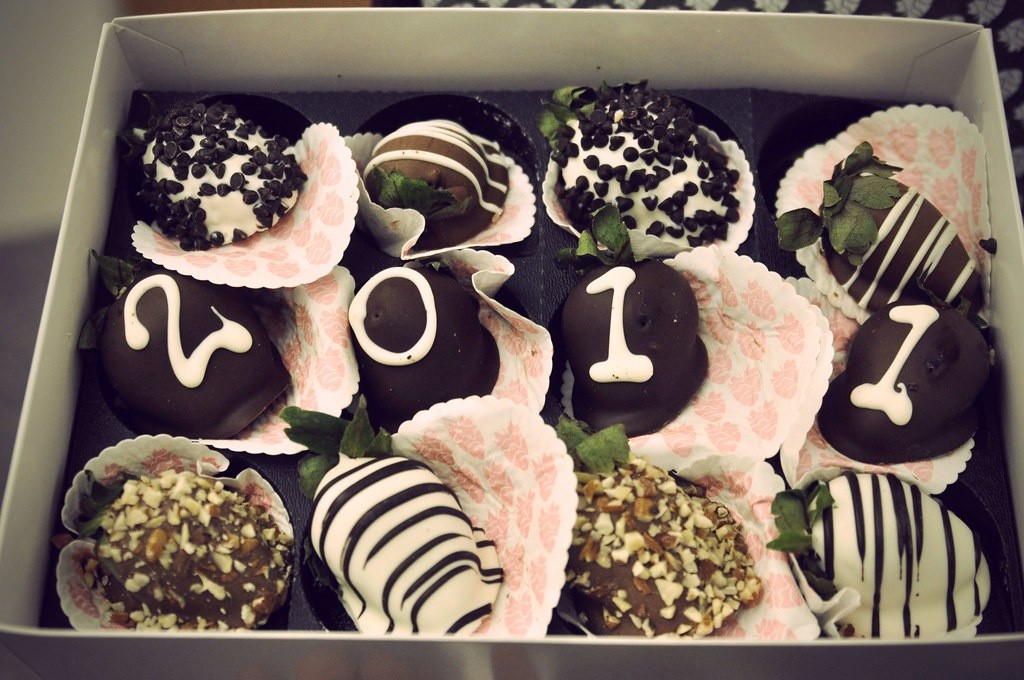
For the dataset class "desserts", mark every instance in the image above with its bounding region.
[60,90,995,643]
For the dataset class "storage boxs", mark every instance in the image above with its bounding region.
[3,6,1024,680]
